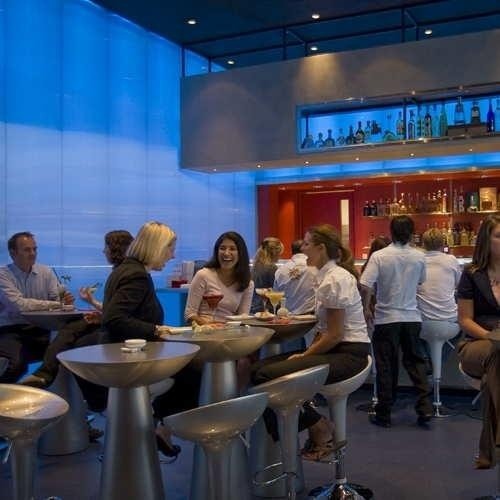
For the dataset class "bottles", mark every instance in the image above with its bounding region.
[361,246,369,259]
[368,219,483,248]
[277,298,288,322]
[363,186,493,216]
[302,97,500,148]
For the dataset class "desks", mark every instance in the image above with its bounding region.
[55,341,201,499]
[22,306,97,455]
[158,326,274,499]
[154,287,191,326]
[230,314,320,494]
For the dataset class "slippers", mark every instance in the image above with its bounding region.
[297,437,332,454]
[301,440,348,461]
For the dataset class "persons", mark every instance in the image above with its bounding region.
[0,232,104,442]
[251,237,319,359]
[98,221,182,458]
[254,224,370,462]
[453,215,499,471]
[184,231,254,390]
[359,214,438,430]
[19,230,135,412]
[355,228,465,412]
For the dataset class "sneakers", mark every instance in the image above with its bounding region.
[416,417,431,429]
[88,428,105,442]
[18,376,46,388]
[369,413,390,428]
[154,422,175,458]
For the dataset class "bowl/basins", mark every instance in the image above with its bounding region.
[64,304,74,312]
[124,339,147,349]
[227,321,241,327]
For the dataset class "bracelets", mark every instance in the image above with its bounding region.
[95,301,101,309]
[155,325,164,335]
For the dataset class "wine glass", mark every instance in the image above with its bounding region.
[202,291,224,327]
[56,279,67,312]
[255,288,272,318]
[265,291,285,322]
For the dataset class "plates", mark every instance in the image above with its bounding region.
[225,315,254,320]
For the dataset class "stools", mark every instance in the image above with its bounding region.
[162,391,269,499]
[306,355,374,499]
[418,320,462,418]
[0,384,71,500]
[355,323,401,415]
[246,364,333,500]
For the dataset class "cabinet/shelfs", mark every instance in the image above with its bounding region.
[297,82,500,153]
[362,206,497,253]
